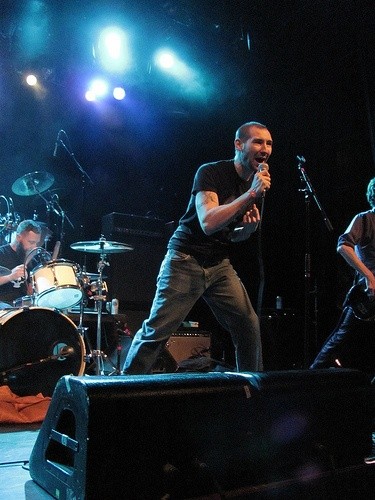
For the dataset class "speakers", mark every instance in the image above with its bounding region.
[162,332,212,363]
[29,371,265,500]
[100,212,166,308]
[227,367,367,484]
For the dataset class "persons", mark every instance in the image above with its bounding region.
[118,122,273,376]
[306,178,375,369]
[0,219,42,308]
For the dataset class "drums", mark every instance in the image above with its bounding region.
[30,258,83,310]
[0,305,87,398]
[13,295,33,306]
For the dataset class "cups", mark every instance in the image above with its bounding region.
[276,296,282,309]
[111,299,119,314]
[106,301,111,312]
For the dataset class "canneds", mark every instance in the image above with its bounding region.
[106,299,118,314]
[276,296,282,309]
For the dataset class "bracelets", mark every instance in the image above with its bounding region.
[246,188,256,198]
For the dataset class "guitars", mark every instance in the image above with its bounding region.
[348,276,375,319]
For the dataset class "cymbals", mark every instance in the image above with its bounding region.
[71,241,135,254]
[82,271,108,279]
[0,265,12,277]
[12,170,55,195]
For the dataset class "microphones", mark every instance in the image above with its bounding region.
[53,132,61,157]
[60,346,75,356]
[259,162,270,192]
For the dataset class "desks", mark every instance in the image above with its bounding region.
[63,312,127,375]
